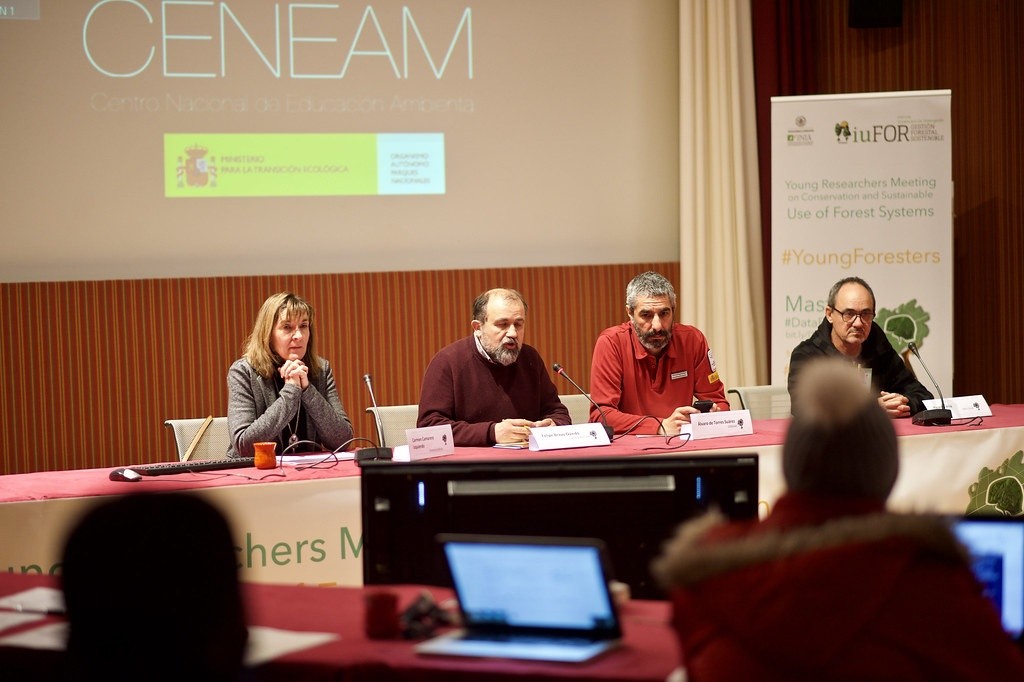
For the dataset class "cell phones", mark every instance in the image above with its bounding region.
[689,401,713,416]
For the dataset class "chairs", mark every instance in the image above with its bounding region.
[162,417,231,463]
[727,384,791,419]
[367,403,419,448]
[558,394,591,425]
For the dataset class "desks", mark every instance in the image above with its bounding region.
[0,404,1024,682]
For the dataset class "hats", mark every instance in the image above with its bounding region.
[779,361,900,505]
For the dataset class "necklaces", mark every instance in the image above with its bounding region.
[273,372,301,449]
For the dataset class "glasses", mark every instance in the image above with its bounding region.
[832,307,876,324]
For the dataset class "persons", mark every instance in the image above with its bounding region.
[56,491,250,682]
[588,270,730,436]
[417,288,571,447]
[225,288,354,459]
[649,354,1024,682]
[787,277,934,418]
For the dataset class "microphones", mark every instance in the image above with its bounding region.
[907,342,952,427]
[551,363,614,443]
[353,373,393,463]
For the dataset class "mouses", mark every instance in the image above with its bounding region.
[109,468,143,482]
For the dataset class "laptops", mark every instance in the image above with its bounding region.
[413,531,625,663]
[944,514,1024,641]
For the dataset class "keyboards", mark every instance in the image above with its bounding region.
[128,456,257,477]
